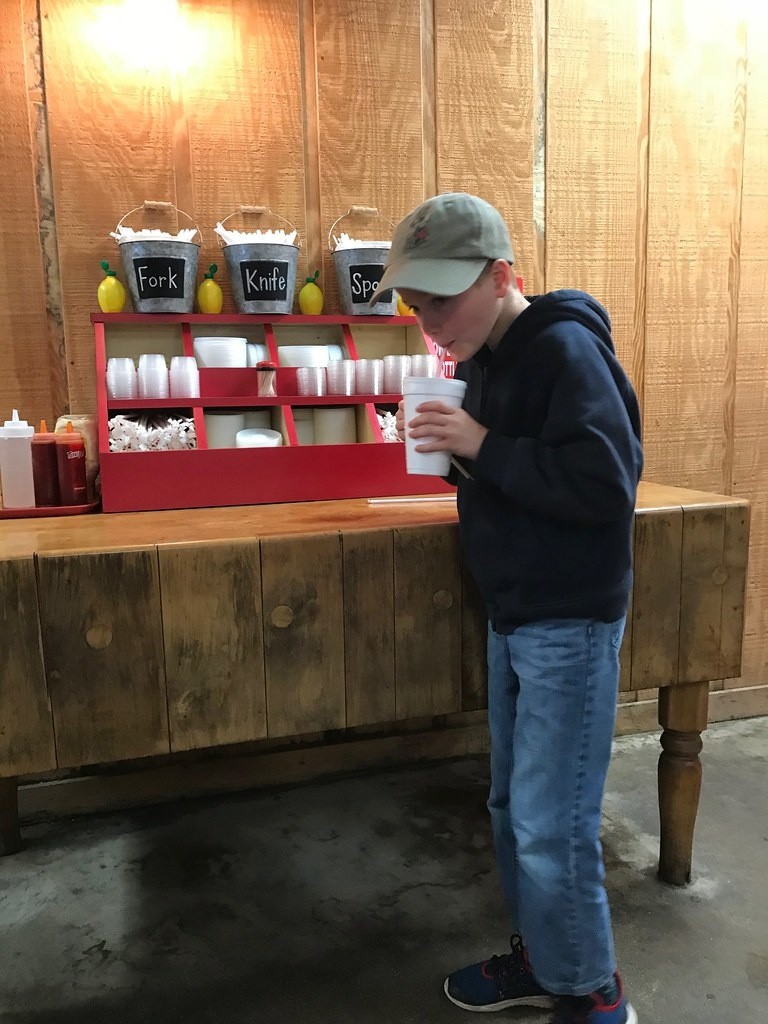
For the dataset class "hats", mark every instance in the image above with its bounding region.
[367,192,513,307]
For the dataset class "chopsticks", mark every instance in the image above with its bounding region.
[108,413,197,452]
[376,408,403,442]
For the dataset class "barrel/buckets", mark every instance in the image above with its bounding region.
[328,206,397,316]
[114,200,202,314]
[216,205,301,314]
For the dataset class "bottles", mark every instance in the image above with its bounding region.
[197,264,223,314]
[256,361,277,397]
[0,409,36,509]
[299,269,323,316]
[31,420,59,506]
[397,295,415,316]
[55,421,87,506]
[96,260,126,313]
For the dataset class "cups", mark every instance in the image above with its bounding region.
[402,376,468,476]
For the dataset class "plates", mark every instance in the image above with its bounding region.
[291,407,357,446]
[193,337,344,368]
[203,410,283,449]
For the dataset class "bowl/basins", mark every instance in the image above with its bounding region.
[296,354,437,397]
[105,353,200,399]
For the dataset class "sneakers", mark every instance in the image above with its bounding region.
[445,934,560,1013]
[550,969,638,1024]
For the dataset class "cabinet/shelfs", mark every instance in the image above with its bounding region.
[89,311,459,515]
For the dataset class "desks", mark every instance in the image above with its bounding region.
[0,480,750,885]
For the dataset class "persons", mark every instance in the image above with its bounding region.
[369,193,644,1024]
